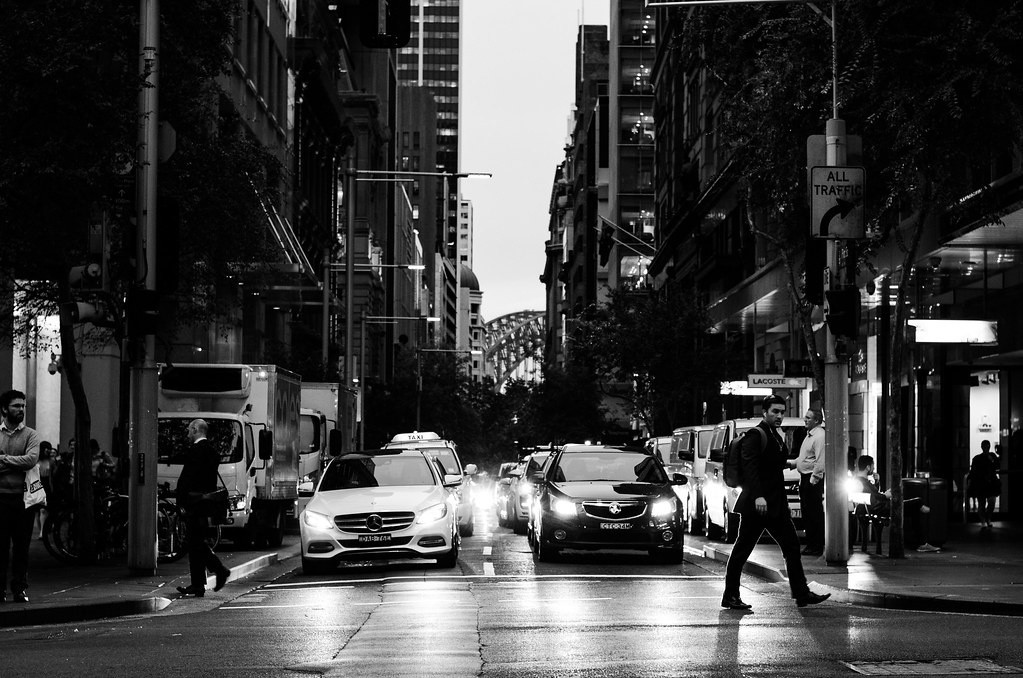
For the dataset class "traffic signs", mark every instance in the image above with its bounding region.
[810,165,866,239]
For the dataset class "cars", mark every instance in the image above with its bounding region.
[489,442,562,536]
[298,449,463,575]
[527,440,685,564]
[380,431,478,537]
[645,435,688,522]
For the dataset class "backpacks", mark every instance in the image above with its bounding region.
[723,427,766,488]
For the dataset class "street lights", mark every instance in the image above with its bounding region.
[415,336,483,431]
[323,249,425,386]
[361,311,441,451]
[344,152,415,447]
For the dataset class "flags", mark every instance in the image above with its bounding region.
[599,221,615,256]
[600,238,615,267]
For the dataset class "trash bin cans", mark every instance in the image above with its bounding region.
[902,477,946,549]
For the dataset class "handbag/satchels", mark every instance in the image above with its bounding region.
[202,486,230,513]
[23,464,47,508]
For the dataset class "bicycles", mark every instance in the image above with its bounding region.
[43,473,222,566]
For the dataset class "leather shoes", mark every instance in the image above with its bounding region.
[720,595,753,610]
[794,592,831,607]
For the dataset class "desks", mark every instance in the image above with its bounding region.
[900,477,951,553]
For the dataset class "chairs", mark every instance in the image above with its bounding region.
[847,477,891,555]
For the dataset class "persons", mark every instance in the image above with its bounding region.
[783,407,826,555]
[37,438,116,539]
[175,418,231,595]
[721,395,831,608]
[851,454,941,552]
[0,389,40,605]
[968,441,1002,528]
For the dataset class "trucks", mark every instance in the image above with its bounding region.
[287,383,358,525]
[157,364,300,551]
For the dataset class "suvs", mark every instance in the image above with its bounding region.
[670,424,718,534]
[701,417,857,540]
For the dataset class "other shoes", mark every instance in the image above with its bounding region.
[800,547,823,556]
[918,543,940,552]
[0,590,7,602]
[13,588,28,602]
[213,566,230,592]
[176,585,206,597]
